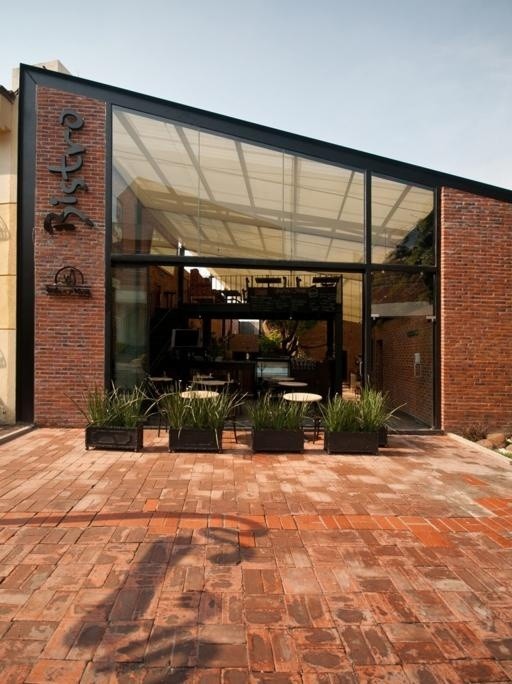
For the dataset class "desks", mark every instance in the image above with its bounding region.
[145,373,323,444]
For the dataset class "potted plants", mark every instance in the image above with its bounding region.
[317,374,408,454]
[63,377,144,452]
[242,389,312,452]
[144,375,242,453]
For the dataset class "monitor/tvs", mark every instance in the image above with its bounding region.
[172,329,200,346]
